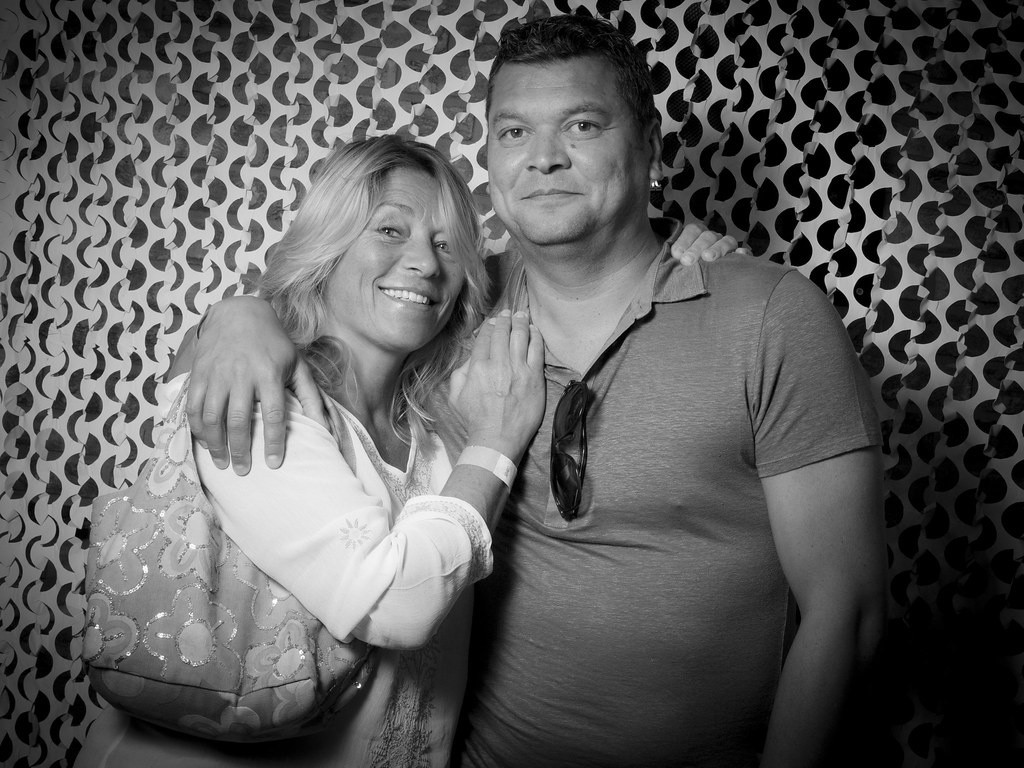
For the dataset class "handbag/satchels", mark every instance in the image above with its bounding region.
[83,378,379,750]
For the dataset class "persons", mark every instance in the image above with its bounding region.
[76,134,754,767]
[167,13,888,767]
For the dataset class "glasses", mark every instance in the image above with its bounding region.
[550,379,596,521]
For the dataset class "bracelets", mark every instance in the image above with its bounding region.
[456,446,517,491]
